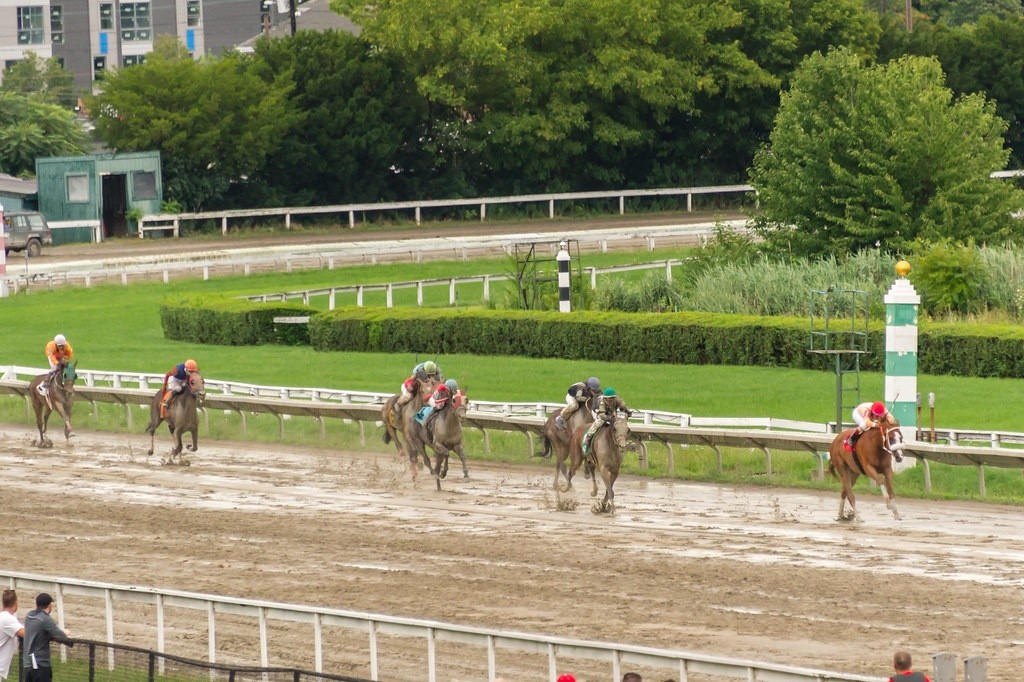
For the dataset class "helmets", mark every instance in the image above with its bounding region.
[185,360,198,370]
[424,361,436,374]
[445,379,458,394]
[871,402,884,416]
[603,387,617,399]
[587,377,600,392]
[55,334,65,347]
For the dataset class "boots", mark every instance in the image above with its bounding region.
[43,371,52,383]
[164,390,178,406]
[394,396,405,412]
[584,433,593,446]
[847,430,860,446]
[423,410,437,425]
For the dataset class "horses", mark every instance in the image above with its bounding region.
[381,375,470,491]
[828,420,905,520]
[28,357,78,443]
[146,368,206,464]
[531,394,631,513]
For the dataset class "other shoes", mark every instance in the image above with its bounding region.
[556,416,563,430]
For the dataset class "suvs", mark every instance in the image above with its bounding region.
[3,211,53,257]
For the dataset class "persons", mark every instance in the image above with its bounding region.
[42,335,74,387]
[393,361,442,411]
[22,593,68,682]
[623,672,642,682]
[847,402,893,446]
[160,359,198,408]
[554,377,599,430]
[422,379,458,426]
[888,651,930,682]
[0,589,25,682]
[584,389,630,451]
